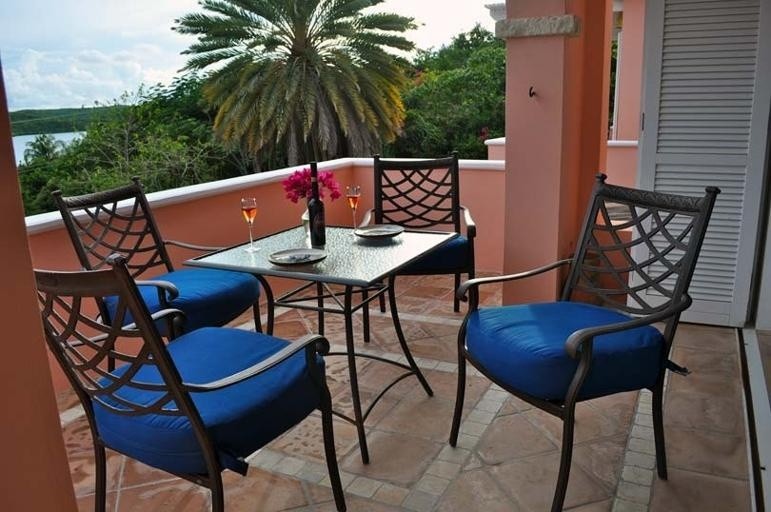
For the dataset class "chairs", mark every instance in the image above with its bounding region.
[448,174,721,512]
[359,151,476,343]
[52,176,261,375]
[33,253,347,512]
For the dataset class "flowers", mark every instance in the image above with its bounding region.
[281,168,341,203]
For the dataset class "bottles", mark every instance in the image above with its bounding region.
[307,161,325,246]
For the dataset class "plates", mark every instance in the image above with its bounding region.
[354,223,406,240]
[266,248,327,266]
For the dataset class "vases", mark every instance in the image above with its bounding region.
[302,197,310,243]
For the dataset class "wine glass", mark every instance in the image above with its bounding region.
[241,197,262,253]
[346,185,361,236]
